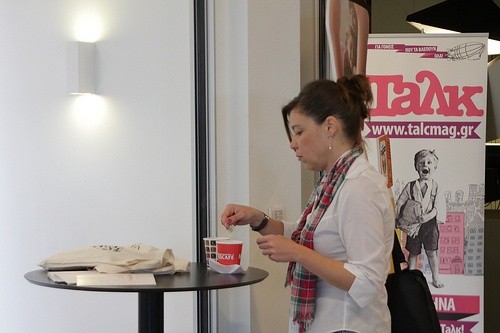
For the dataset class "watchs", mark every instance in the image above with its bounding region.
[250,213,269,232]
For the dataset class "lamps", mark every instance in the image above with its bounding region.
[406,0,500,56]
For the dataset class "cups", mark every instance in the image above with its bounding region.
[203,237,230,267]
[216,240,242,265]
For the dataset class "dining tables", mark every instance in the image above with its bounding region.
[24,262,269,333]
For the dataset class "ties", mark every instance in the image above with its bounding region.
[421,183,428,197]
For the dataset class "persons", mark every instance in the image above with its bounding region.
[220,74,394,333]
[393,149,443,288]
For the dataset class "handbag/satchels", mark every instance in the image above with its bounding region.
[397,199,423,237]
[386,268,444,333]
[38,245,177,276]
